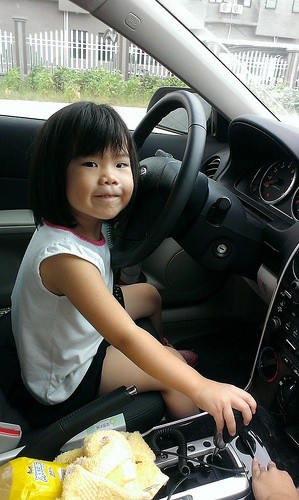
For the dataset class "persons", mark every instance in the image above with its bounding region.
[251,458,299,500]
[10,101,258,436]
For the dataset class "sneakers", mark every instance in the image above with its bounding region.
[162,338,198,368]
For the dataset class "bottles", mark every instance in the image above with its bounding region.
[0,457,73,500]
